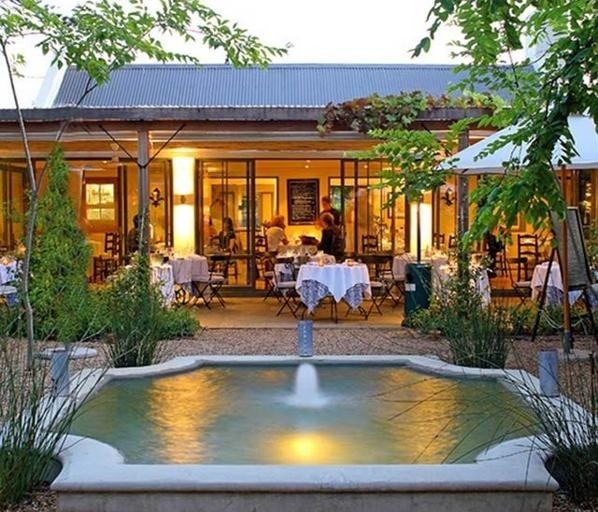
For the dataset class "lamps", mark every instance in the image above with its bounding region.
[440,188,457,206]
[148,188,164,206]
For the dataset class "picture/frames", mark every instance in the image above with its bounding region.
[511,210,527,234]
[386,191,406,219]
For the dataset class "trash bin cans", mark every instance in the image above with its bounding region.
[401,263,431,327]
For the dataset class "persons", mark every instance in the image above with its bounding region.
[319,195,342,228]
[210,217,236,286]
[265,215,296,281]
[125,214,155,254]
[314,212,344,261]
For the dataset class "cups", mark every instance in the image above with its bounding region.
[318,258,324,267]
[347,260,354,267]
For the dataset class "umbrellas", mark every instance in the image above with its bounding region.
[430,108,598,353]
